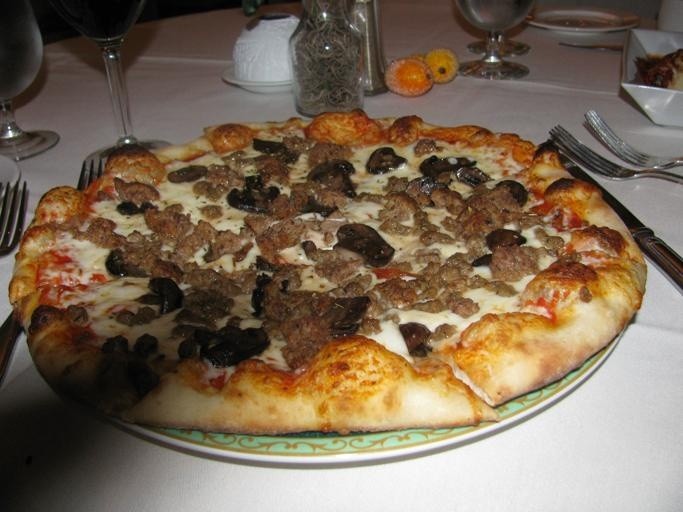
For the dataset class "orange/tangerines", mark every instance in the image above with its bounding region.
[383,58,433,98]
[423,48,459,84]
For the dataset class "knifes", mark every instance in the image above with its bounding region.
[552,147,682,305]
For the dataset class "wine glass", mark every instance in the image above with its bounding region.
[455,1,534,81]
[60,1,172,163]
[0,2,61,164]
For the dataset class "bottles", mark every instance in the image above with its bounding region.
[288,1,363,118]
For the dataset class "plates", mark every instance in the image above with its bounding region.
[84,322,626,467]
[220,67,294,95]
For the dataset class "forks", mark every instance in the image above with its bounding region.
[0,181,32,258]
[543,108,683,192]
[76,157,103,199]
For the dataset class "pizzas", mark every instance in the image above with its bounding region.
[9,109,646,437]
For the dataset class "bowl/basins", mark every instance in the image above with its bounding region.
[620,24,683,128]
[522,5,640,48]
